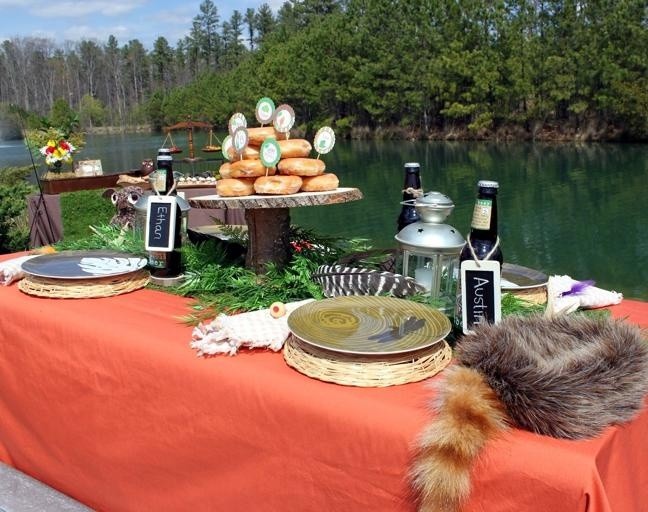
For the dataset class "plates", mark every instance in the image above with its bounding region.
[286,295,452,358]
[500,262,551,291]
[21,250,148,280]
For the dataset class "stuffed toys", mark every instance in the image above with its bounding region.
[100,185,143,229]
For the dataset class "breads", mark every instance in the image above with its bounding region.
[216,126,340,196]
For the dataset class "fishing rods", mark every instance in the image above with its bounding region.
[10,99,55,249]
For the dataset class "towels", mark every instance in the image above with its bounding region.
[547,272,621,316]
[185,298,317,357]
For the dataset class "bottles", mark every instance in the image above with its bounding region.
[148,149,182,278]
[455,179,503,338]
[394,163,425,230]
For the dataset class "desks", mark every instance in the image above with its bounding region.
[31,168,144,245]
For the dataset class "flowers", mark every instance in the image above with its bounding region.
[25,123,85,166]
